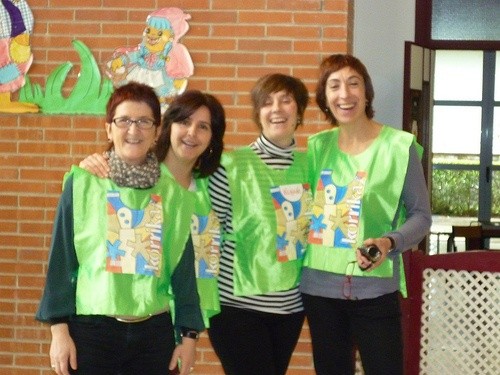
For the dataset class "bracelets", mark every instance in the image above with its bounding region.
[180,326,199,340]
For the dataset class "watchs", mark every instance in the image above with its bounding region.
[384,236,396,255]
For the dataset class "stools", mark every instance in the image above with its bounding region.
[429,225,454,256]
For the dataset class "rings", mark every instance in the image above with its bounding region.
[50,364,56,369]
[189,366,194,372]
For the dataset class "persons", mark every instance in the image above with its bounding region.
[77,90,226,375]
[207,73,315,375]
[34,81,206,375]
[298,54,433,375]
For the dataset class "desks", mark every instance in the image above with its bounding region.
[451,225,500,251]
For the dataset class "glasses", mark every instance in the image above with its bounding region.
[110,116,157,129]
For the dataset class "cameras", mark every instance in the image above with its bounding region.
[358,245,382,263]
